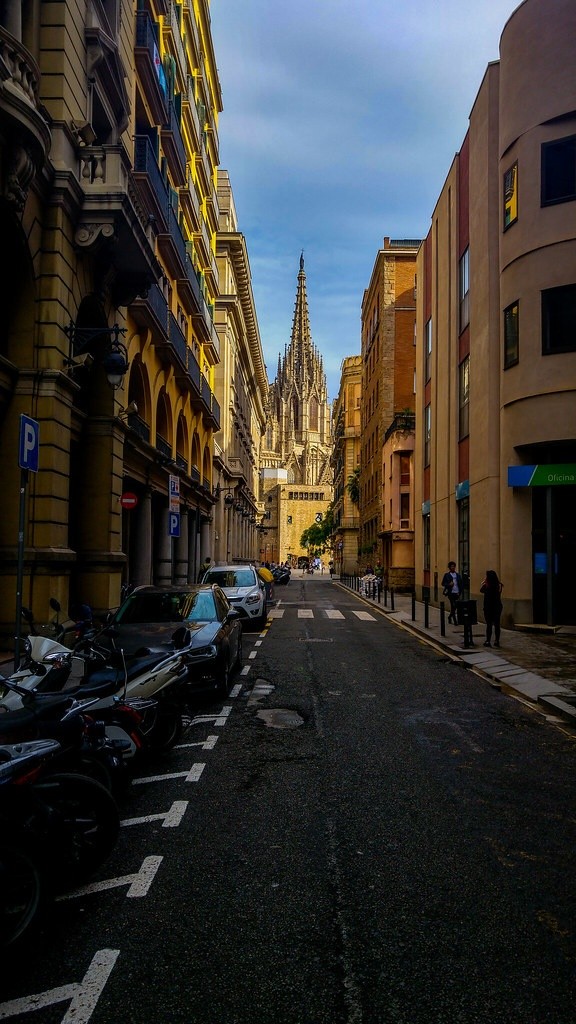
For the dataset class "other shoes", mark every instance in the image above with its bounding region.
[493,642,500,647]
[454,620,457,626]
[483,642,491,646]
[448,617,452,624]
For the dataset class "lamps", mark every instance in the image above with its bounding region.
[233,497,243,517]
[163,458,175,468]
[71,120,97,146]
[63,320,130,390]
[241,505,268,537]
[126,400,139,415]
[212,485,235,509]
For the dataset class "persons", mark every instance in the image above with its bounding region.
[261,560,289,571]
[480,569,504,647]
[200,557,213,582]
[441,561,464,626]
[374,561,384,592]
[363,563,374,576]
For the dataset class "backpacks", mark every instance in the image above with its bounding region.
[202,564,210,578]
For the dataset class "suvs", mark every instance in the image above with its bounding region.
[95,582,243,698]
[200,563,268,631]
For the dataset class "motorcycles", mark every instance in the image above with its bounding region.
[259,560,291,586]
[0,596,196,955]
